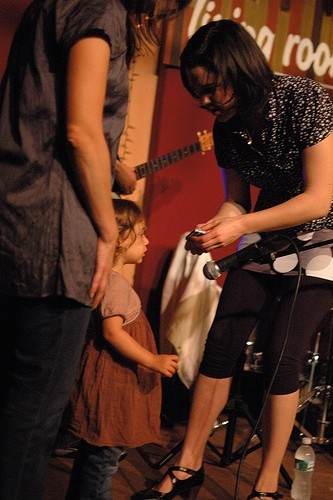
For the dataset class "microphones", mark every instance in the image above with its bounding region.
[203,230,296,280]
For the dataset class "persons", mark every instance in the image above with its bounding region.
[0,0,192,500]
[133,19,333,500]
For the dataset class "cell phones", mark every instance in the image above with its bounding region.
[186,228,208,240]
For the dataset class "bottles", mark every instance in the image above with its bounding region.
[290,437,316,500]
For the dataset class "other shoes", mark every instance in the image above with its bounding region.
[52,434,127,462]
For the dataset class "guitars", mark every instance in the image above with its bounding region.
[132,130,214,181]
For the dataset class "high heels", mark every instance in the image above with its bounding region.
[246,491,282,500]
[132,465,205,499]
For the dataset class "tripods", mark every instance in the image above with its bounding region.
[154,309,333,489]
